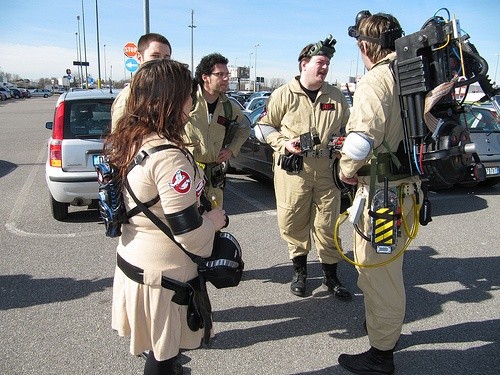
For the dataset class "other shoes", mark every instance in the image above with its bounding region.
[171,364,183,375]
[139,351,149,361]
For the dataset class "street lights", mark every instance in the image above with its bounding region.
[494,53,500,82]
[248,52,254,68]
[103,45,106,87]
[76,15,83,89]
[254,43,260,92]
[75,32,80,87]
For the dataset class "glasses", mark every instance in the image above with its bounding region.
[210,72,231,77]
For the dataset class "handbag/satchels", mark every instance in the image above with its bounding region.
[198,232,243,287]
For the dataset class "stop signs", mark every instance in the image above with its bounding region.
[123,42,138,58]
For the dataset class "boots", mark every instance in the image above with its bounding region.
[364,320,397,350]
[338,345,395,375]
[291,256,308,297]
[321,261,352,301]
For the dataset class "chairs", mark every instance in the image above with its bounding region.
[74,108,93,126]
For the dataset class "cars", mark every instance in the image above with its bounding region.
[45,88,126,221]
[0,82,500,193]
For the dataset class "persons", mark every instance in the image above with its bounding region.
[179,54,250,210]
[337,12,425,375]
[110,33,172,133]
[254,35,353,303]
[102,58,227,374]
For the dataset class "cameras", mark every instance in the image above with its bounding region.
[198,201,229,228]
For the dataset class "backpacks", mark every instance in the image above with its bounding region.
[98,145,191,238]
